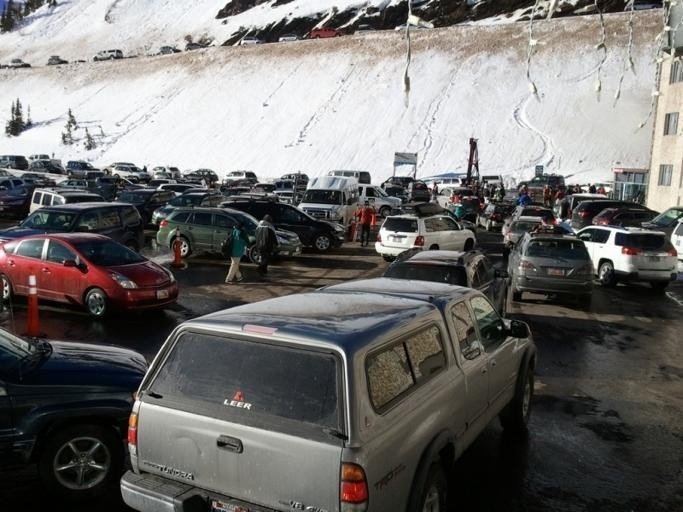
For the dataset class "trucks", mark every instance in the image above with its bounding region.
[116,277,539,512]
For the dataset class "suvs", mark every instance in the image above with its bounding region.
[386,249,509,317]
[427,172,683,306]
[216,198,334,253]
[0,201,145,253]
[156,206,303,264]
[0,152,401,230]
[371,205,479,259]
[379,176,429,204]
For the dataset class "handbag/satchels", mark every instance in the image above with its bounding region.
[220,234,232,257]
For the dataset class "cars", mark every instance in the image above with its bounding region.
[47,56,69,64]
[624,1,658,10]
[309,27,344,38]
[279,33,301,42]
[185,43,206,51]
[1,59,28,68]
[394,24,418,31]
[0,233,180,317]
[156,46,179,56]
[240,38,266,46]
[0,328,147,501]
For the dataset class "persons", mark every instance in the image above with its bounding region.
[432,182,439,195]
[540,214,550,227]
[446,176,610,220]
[254,214,279,275]
[224,219,252,286]
[351,199,378,248]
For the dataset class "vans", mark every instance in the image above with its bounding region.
[92,49,122,60]
